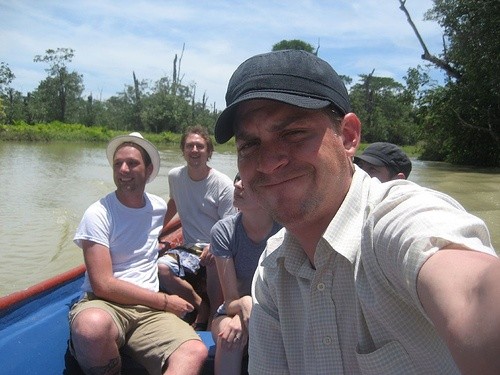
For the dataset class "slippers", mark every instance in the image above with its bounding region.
[190,322,208,331]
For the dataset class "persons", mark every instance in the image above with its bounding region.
[209,172,284,374]
[213,49,500,374]
[68,132,209,375]
[157,125,238,331]
[351,142,413,184]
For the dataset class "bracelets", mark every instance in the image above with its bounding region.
[163,293,167,311]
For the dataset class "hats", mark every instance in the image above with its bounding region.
[215,50,351,144]
[106,132,160,183]
[352,142,412,179]
